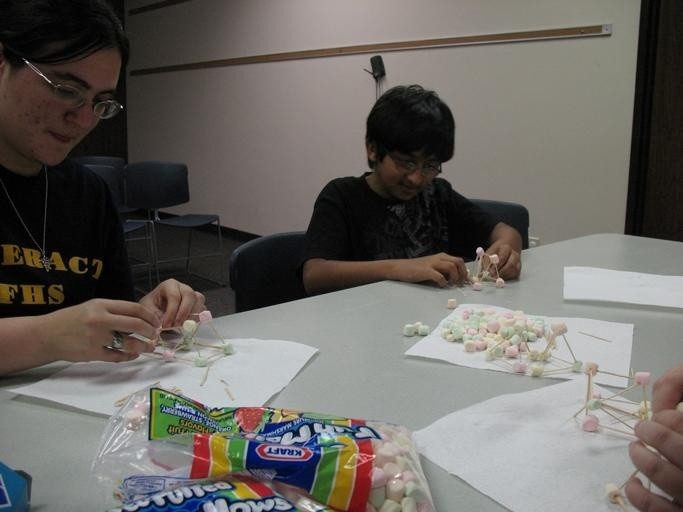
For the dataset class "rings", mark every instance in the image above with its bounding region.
[106,332,124,352]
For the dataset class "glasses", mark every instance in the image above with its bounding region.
[20,57,125,120]
[386,149,441,178]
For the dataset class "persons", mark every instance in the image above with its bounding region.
[623,365,683,512]
[0,0,209,376]
[294,85,523,296]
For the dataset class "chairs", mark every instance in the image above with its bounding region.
[463,198,529,248]
[124,161,225,289]
[230,231,311,312]
[80,164,155,290]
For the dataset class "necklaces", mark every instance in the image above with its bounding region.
[1,164,52,272]
